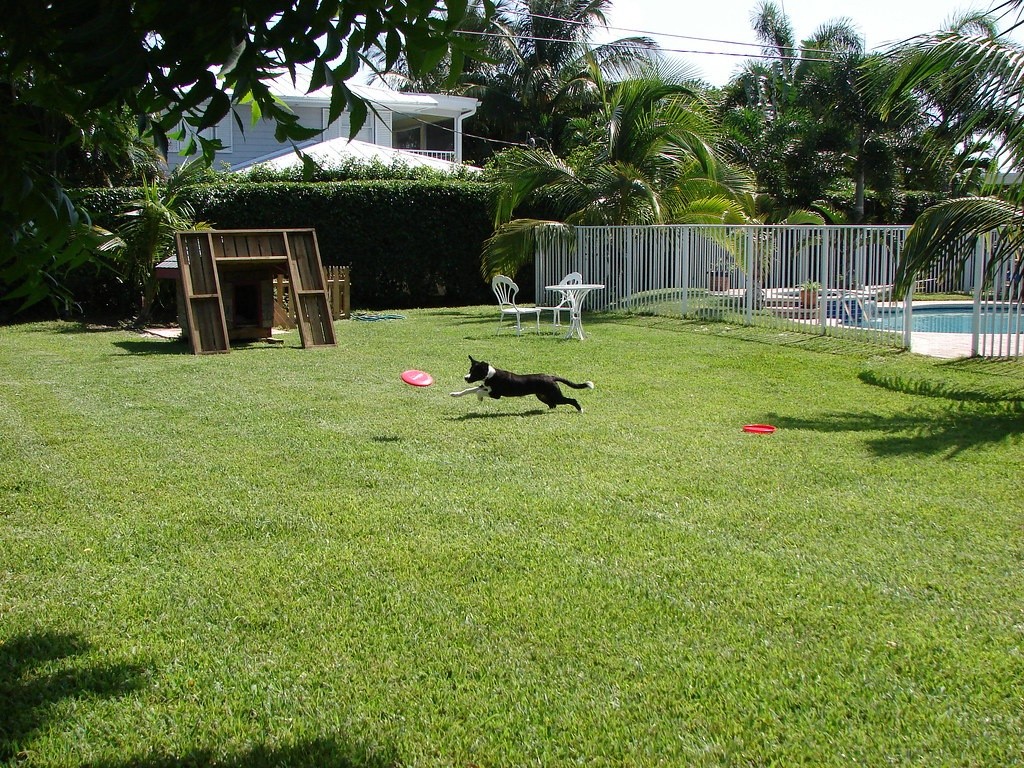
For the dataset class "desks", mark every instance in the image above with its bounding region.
[546,284,604,339]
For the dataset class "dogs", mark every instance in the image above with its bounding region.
[450,354,595,415]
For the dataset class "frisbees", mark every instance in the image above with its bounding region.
[398,367,435,389]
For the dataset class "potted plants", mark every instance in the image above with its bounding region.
[707,258,735,289]
[796,278,822,308]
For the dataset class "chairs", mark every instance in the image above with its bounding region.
[536,271,583,335]
[492,275,543,338]
